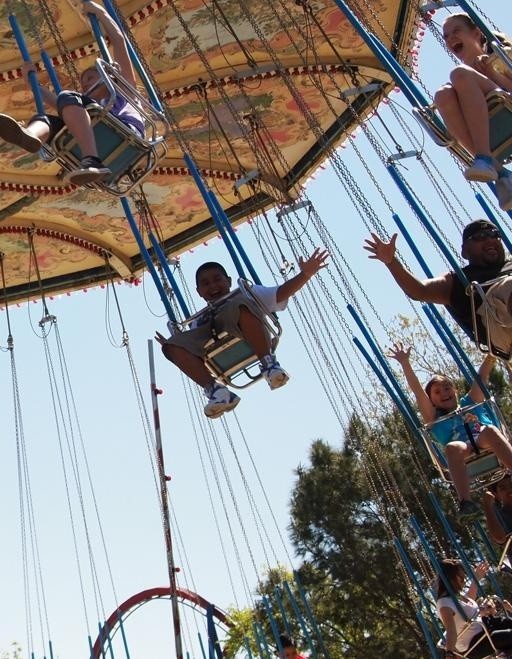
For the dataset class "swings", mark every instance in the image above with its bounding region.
[2,2,509,657]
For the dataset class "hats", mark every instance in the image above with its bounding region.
[463,220,501,242]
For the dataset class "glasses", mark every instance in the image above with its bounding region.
[467,230,498,241]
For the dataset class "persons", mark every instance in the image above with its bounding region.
[0,1,146,186]
[434,14,512,212]
[274,635,307,659]
[436,559,512,659]
[155,247,330,418]
[387,342,512,526]
[363,219,512,360]
[482,474,512,568]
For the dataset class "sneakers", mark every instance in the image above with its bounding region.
[464,158,498,182]
[66,157,114,188]
[0,113,43,154]
[496,169,512,210]
[457,499,483,527]
[259,353,290,391]
[203,383,241,419]
